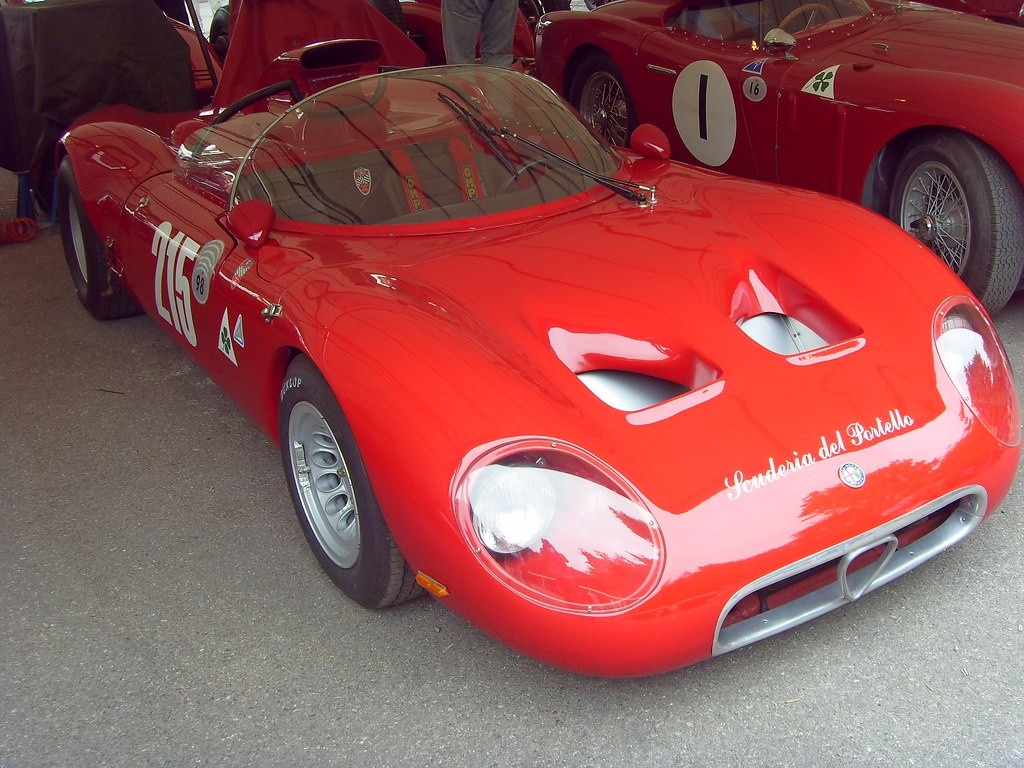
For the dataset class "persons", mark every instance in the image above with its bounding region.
[440,0,518,117]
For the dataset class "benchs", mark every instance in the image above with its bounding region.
[239,121,514,223]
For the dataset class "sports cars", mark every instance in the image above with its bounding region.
[530,0,1024,317]
[46,0,1024,679]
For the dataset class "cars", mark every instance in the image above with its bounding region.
[1,0,549,221]
